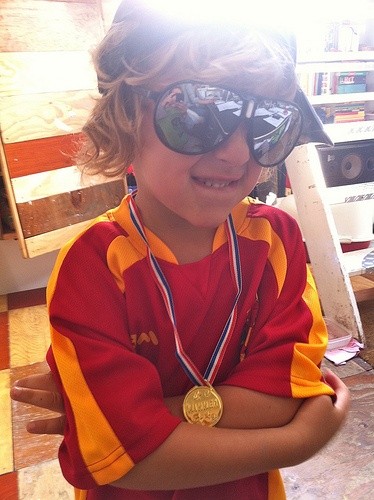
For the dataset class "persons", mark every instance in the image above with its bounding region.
[160,83,219,152]
[4,0,352,500]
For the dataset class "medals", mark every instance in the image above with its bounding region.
[182,384,222,429]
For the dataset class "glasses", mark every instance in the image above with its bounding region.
[120,78,304,167]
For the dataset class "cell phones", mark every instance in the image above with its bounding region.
[176,93,184,103]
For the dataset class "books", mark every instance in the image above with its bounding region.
[311,18,368,123]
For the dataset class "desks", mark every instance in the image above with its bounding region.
[1,239,60,475]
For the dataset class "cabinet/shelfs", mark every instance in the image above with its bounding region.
[284,48,374,348]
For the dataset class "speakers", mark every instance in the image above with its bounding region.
[316,140,374,187]
